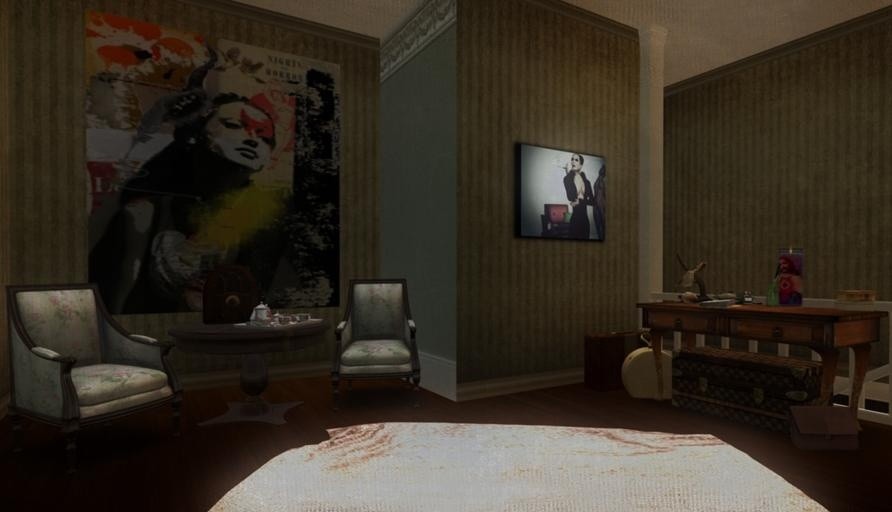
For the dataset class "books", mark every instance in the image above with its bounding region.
[699,299,738,308]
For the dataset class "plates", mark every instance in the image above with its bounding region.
[310,319,322,324]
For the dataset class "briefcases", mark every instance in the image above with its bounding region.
[584,329,649,391]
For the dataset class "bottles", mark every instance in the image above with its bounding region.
[672,319,682,352]
[742,290,753,304]
[774,247,809,306]
[766,271,780,305]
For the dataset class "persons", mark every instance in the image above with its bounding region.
[563,154,593,237]
[774,258,802,305]
[90,92,277,315]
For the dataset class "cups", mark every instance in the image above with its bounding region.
[273,314,309,325]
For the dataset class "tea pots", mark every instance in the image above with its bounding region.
[253,300,272,325]
